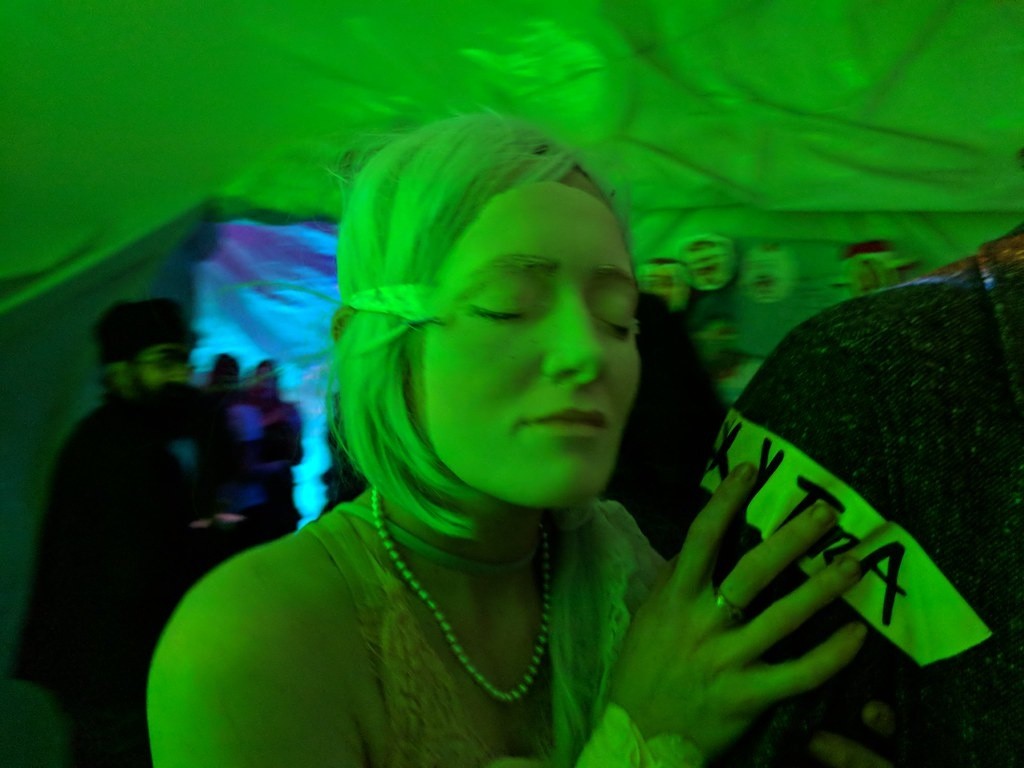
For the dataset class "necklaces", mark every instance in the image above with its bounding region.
[371,488,551,701]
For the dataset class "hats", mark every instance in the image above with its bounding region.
[96,297,200,365]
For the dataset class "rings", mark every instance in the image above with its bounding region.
[712,589,745,615]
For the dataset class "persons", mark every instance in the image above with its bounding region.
[146,111,866,768]
[603,292,729,559]
[697,220,1024,768]
[320,409,366,516]
[15,298,300,768]
[208,351,303,467]
